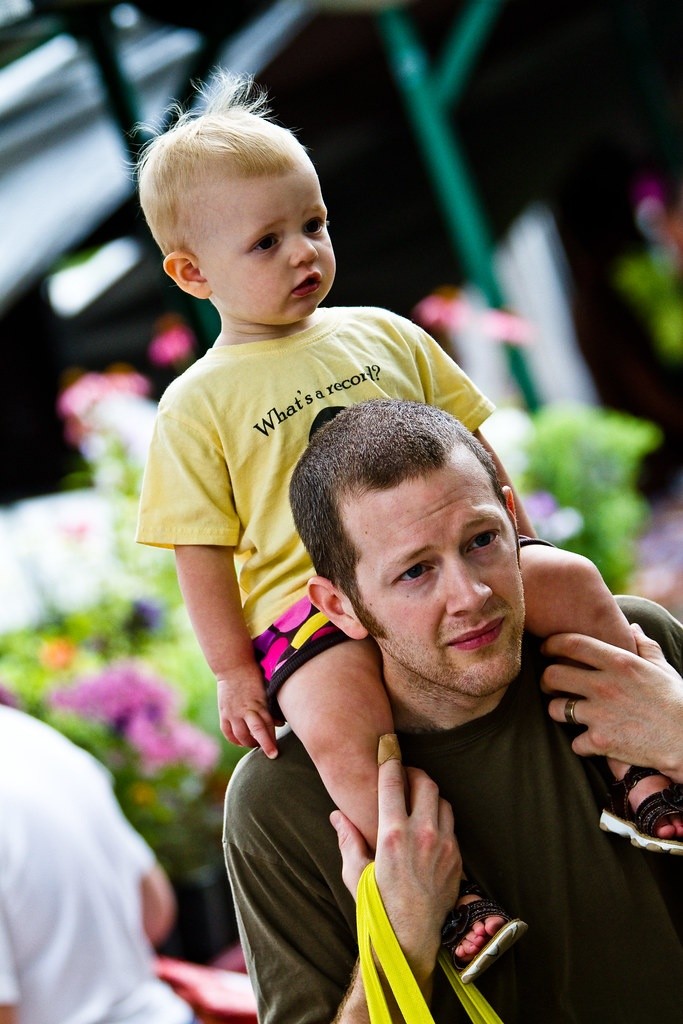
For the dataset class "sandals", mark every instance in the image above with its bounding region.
[443,881,527,984]
[597,769,683,856]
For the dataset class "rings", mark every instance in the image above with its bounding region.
[564,697,580,726]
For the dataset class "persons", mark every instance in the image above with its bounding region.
[0,76,683,1024]
